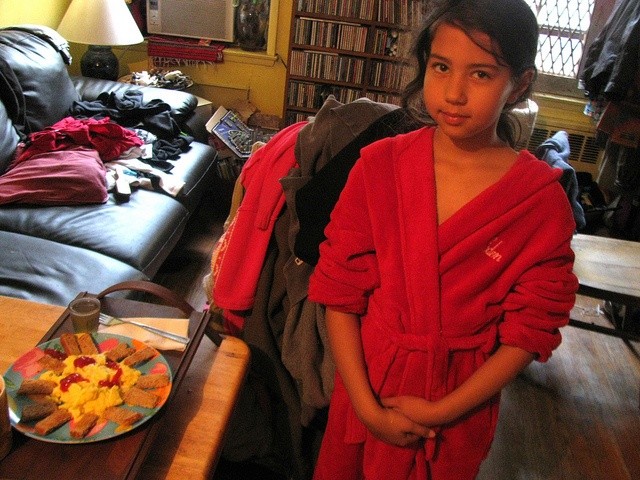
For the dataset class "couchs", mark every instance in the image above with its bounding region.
[1,25,218,307]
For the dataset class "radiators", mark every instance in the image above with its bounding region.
[528,97,604,176]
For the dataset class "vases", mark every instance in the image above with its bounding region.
[235,0,271,51]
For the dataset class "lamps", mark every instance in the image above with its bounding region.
[55,0,147,81]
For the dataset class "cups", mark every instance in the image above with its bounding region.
[68,296,101,331]
[0,375,13,462]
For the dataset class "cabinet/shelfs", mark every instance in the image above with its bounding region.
[280,0,443,126]
[186,95,213,145]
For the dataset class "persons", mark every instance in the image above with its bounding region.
[306,0,579,480]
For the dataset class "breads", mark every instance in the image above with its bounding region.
[60,331,81,356]
[37,353,67,376]
[16,379,57,396]
[125,388,162,408]
[104,406,143,427]
[123,346,156,368]
[69,412,99,439]
[137,374,170,389]
[22,401,58,422]
[78,332,100,355]
[104,342,129,357]
[109,347,136,362]
[34,407,73,436]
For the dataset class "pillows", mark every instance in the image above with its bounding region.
[0,142,109,207]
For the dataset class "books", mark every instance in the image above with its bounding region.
[287,79,401,109]
[285,109,316,126]
[297,0,426,26]
[207,111,282,184]
[289,50,417,91]
[294,16,409,57]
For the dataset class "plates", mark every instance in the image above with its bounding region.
[4,333,174,446]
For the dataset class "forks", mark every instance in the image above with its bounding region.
[98,311,190,346]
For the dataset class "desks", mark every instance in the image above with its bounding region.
[2,295,249,480]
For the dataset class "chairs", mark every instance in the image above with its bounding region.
[98,237,304,470]
[567,230,639,344]
[535,130,622,235]
